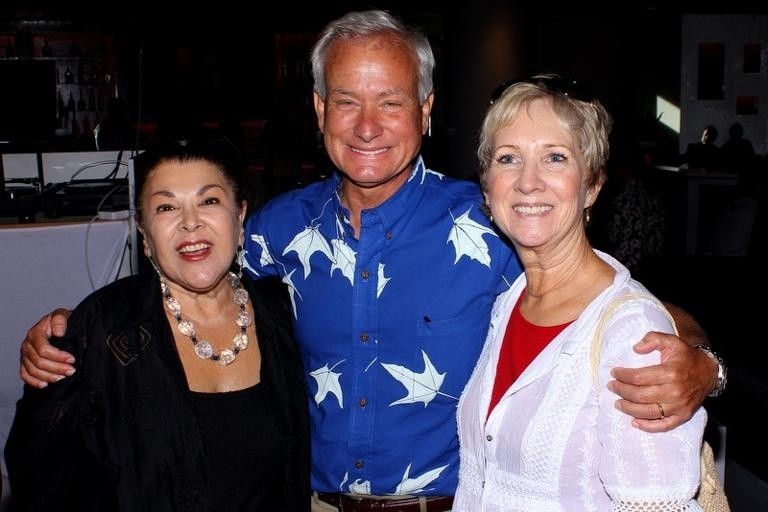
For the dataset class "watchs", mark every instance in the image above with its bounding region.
[696,345,726,398]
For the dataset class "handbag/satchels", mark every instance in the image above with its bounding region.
[697,441,731,512]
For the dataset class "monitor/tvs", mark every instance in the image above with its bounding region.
[656,95,681,134]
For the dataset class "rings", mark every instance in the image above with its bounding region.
[657,403,665,419]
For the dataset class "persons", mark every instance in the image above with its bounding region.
[451,73,709,511]
[3,128,310,511]
[20,11,718,511]
[672,124,753,169]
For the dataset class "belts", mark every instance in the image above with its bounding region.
[311,490,454,512]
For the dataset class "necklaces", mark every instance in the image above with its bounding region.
[158,272,253,365]
